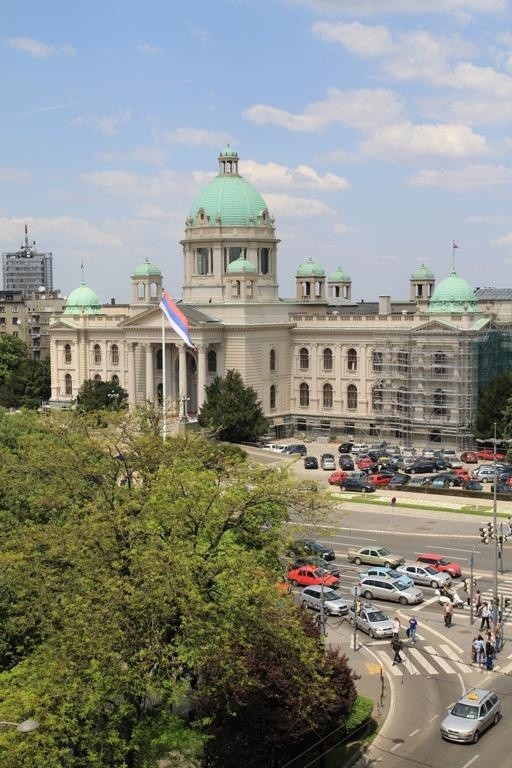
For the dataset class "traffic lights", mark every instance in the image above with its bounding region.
[357,600,363,612]
[464,579,470,593]
[497,535,501,544]
[473,576,477,589]
[487,523,494,539]
[504,596,510,608]
[502,535,507,544]
[479,528,488,544]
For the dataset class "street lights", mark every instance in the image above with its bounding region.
[108,390,120,412]
[476,423,512,648]
[176,394,190,424]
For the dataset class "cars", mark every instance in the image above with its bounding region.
[284,539,461,604]
[328,472,349,485]
[440,688,502,745]
[338,442,512,493]
[303,456,318,470]
[300,585,349,616]
[346,601,396,639]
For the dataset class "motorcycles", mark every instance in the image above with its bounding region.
[435,589,464,609]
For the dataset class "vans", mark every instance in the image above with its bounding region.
[320,453,336,470]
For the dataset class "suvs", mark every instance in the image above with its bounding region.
[262,444,307,456]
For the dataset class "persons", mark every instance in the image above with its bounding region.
[389,580,504,672]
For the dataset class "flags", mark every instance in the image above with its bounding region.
[159,289,195,348]
